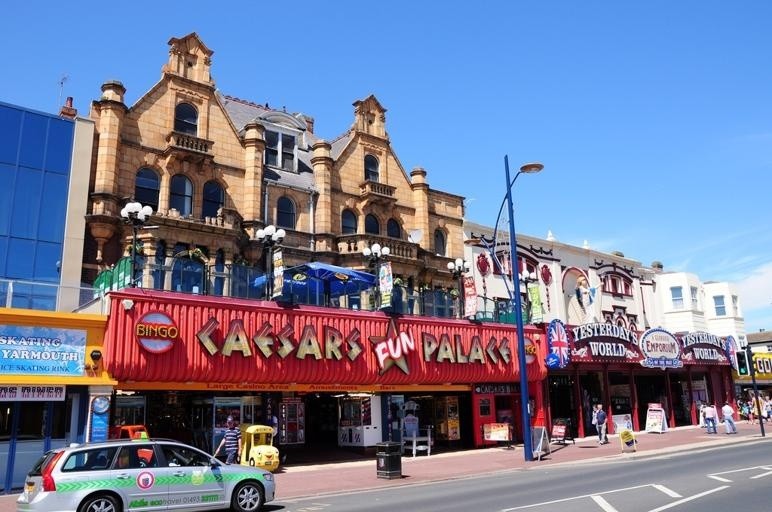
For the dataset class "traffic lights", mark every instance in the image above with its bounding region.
[737,352,748,375]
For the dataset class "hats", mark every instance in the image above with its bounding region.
[227,415,234,423]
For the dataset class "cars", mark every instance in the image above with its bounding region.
[16,439,275,512]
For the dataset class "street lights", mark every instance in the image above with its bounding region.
[447,258,471,320]
[493,164,543,461]
[256,225,286,301]
[363,243,391,312]
[120,202,153,288]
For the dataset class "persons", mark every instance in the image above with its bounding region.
[742,396,772,425]
[216,416,242,465]
[703,403,717,434]
[592,405,601,441]
[596,405,608,445]
[722,401,737,434]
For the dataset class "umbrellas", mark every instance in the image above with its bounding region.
[253,261,379,306]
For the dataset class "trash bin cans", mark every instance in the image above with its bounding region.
[375,442,402,479]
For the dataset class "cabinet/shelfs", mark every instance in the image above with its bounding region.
[401,429,431,457]
